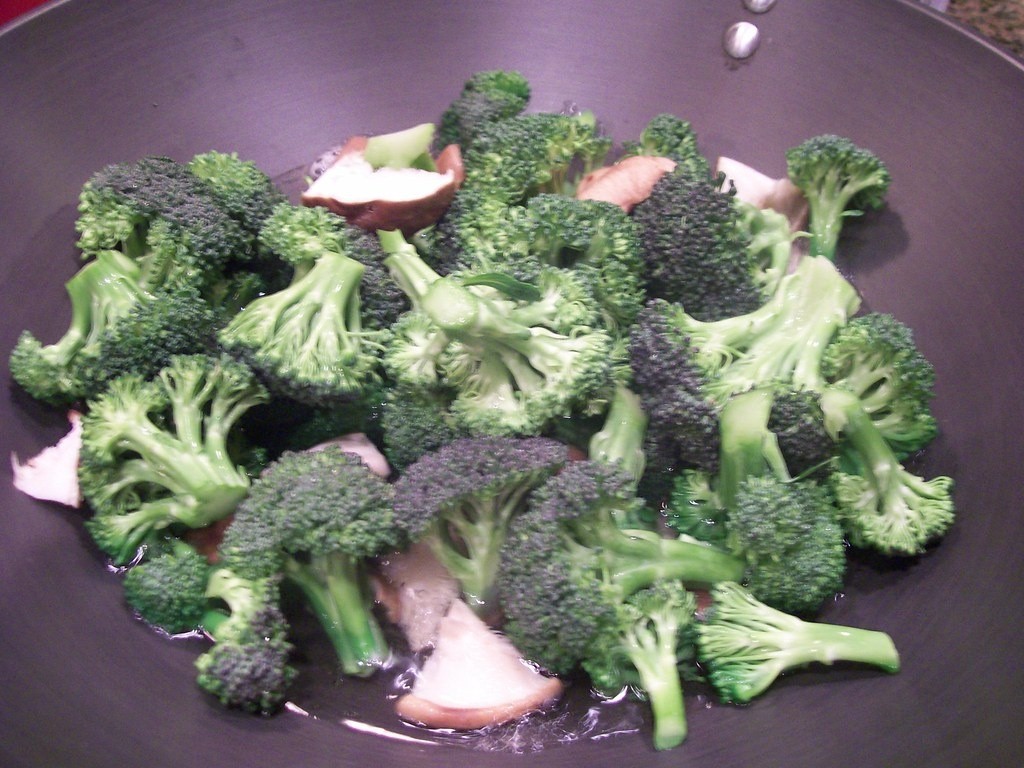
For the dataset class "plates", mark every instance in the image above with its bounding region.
[0,0,1024,768]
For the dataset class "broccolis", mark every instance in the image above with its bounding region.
[11,73,956,747]
